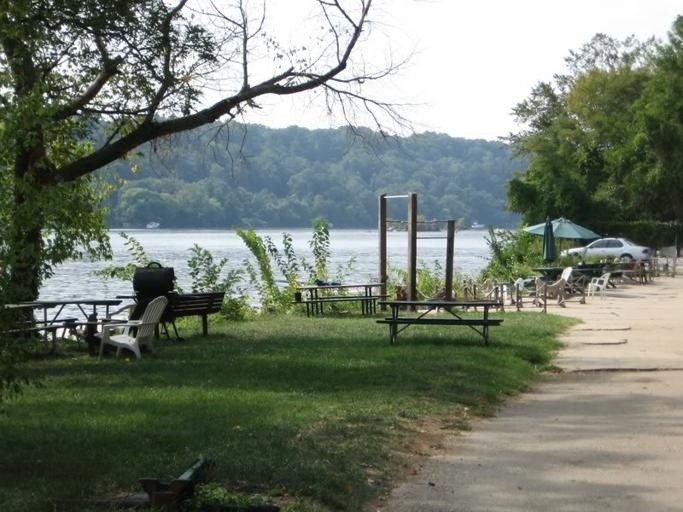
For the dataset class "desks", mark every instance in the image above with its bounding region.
[19,300,122,349]
[296,283,385,316]
[378,300,503,344]
[531,267,562,280]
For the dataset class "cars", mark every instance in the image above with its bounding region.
[559,235,652,266]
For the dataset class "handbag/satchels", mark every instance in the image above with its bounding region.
[133,262,177,293]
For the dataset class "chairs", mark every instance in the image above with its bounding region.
[560,267,574,294]
[94,304,137,355]
[99,296,168,359]
[587,272,610,300]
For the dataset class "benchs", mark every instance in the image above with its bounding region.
[376,318,504,344]
[307,295,390,314]
[385,318,504,322]
[155,292,225,339]
[291,295,391,318]
[2,318,84,354]
[578,264,647,284]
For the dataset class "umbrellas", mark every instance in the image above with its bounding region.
[542,215,558,267]
[522,218,601,267]
